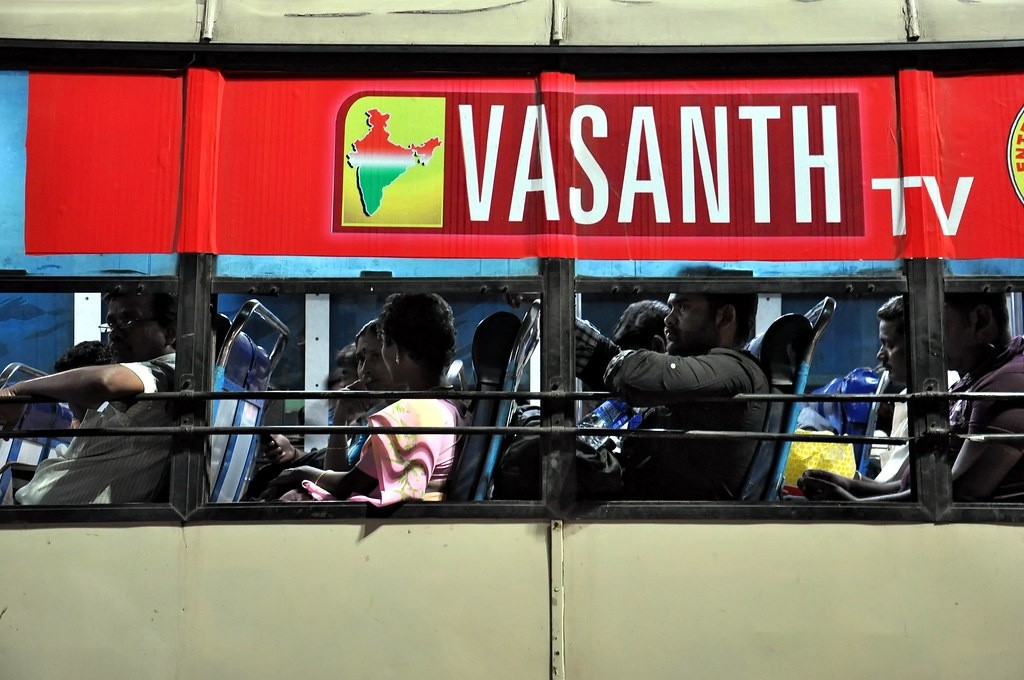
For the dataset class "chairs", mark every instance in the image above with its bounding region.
[0,294,890,503]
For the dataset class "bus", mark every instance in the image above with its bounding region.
[0,1,1024,680]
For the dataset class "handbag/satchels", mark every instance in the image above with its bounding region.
[492,429,622,500]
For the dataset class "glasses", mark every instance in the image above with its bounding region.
[100,317,139,333]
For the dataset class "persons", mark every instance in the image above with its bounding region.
[0,284,174,506]
[497,266,765,501]
[780,290,1024,503]
[245,291,465,506]
